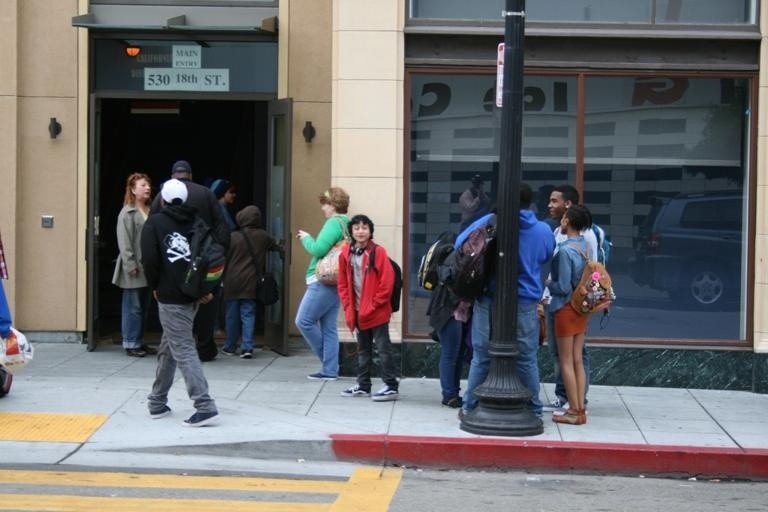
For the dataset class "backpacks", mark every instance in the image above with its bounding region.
[389,256,402,313]
[435,223,496,302]
[570,261,614,317]
[418,229,456,293]
[179,221,228,300]
[597,237,611,265]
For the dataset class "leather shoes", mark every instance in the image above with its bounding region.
[139,344,157,355]
[126,347,146,357]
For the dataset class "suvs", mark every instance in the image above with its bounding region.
[627,193,743,308]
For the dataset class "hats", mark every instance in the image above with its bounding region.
[209,178,232,199]
[171,160,192,174]
[160,177,189,205]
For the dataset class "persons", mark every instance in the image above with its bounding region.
[219,205,278,357]
[141,177,219,425]
[416,173,611,426]
[294,188,351,381]
[148,161,229,360]
[211,179,239,231]
[0,233,17,398]
[111,172,152,357]
[338,215,400,400]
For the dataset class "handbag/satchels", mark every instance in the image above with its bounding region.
[257,242,282,306]
[314,238,351,287]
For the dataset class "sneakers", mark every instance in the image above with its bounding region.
[551,408,587,426]
[182,408,220,428]
[148,405,172,418]
[540,397,564,412]
[221,346,235,356]
[371,385,400,403]
[1,371,12,398]
[552,401,571,416]
[340,382,373,398]
[306,372,339,381]
[239,349,254,358]
[440,393,466,409]
